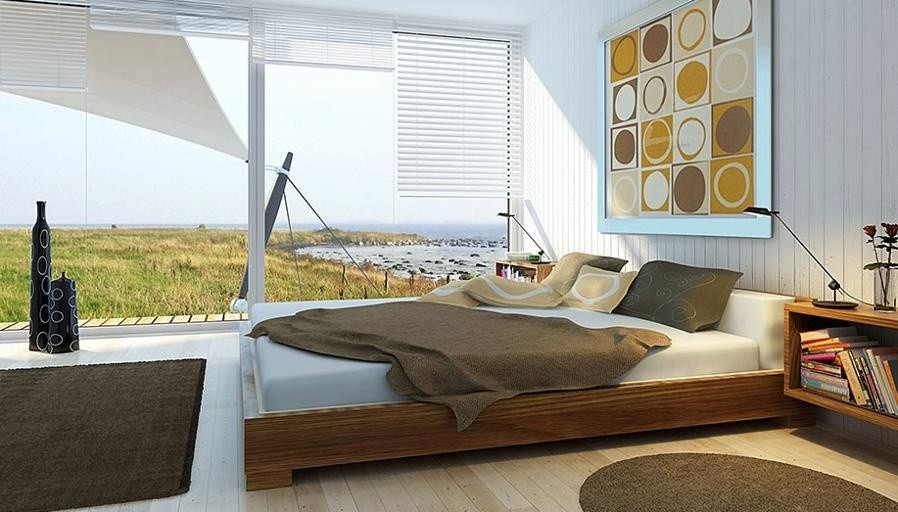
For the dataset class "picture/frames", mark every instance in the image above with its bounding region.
[595,0,775,241]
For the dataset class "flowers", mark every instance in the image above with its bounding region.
[860,220,897,306]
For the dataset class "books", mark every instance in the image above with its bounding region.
[799,325,898,420]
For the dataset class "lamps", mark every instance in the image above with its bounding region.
[496,212,551,265]
[743,206,859,310]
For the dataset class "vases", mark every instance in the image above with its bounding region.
[872,266,897,312]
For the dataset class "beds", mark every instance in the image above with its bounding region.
[237,285,798,493]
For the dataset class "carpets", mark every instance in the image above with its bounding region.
[0,354,207,512]
[578,449,896,512]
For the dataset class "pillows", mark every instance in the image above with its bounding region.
[560,265,638,314]
[612,260,743,333]
[418,280,481,308]
[463,273,571,309]
[540,252,629,296]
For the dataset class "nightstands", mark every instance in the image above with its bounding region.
[781,298,896,431]
